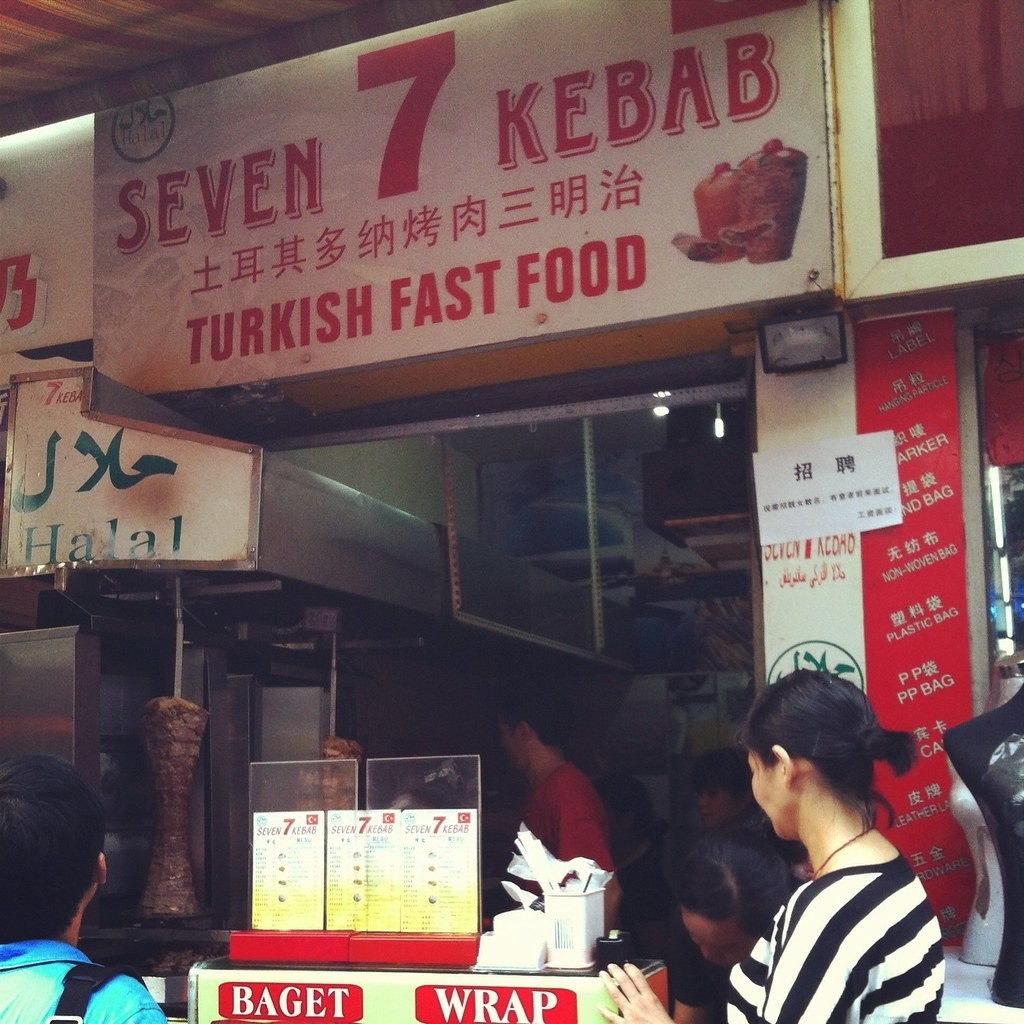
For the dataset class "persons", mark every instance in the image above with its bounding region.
[597,670,947,1024]
[0,759,168,1024]
[382,679,807,1024]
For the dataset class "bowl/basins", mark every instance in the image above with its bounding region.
[596,936,636,962]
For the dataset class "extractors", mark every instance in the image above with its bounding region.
[258,453,642,654]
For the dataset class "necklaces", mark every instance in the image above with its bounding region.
[814,828,876,877]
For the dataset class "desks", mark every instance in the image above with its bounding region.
[189,953,671,1024]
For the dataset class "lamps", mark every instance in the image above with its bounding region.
[989,465,1015,638]
[756,305,850,376]
